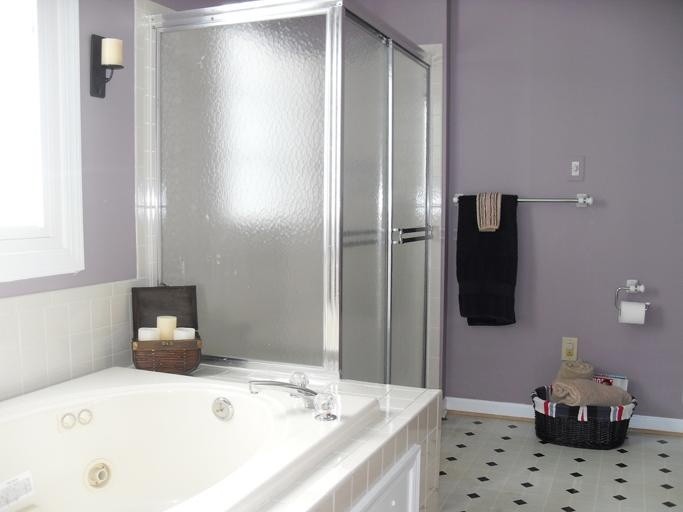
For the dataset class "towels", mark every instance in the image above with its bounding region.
[457,193,517,327]
[551,360,631,409]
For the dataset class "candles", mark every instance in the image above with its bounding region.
[137,314,195,342]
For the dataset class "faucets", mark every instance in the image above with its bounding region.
[248,377,320,409]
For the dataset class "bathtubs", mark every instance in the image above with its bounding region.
[0,363,380,512]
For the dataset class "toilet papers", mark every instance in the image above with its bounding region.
[617,301,647,328]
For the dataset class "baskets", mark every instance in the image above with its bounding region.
[530,383,638,450]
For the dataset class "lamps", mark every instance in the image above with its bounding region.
[90,30,126,99]
